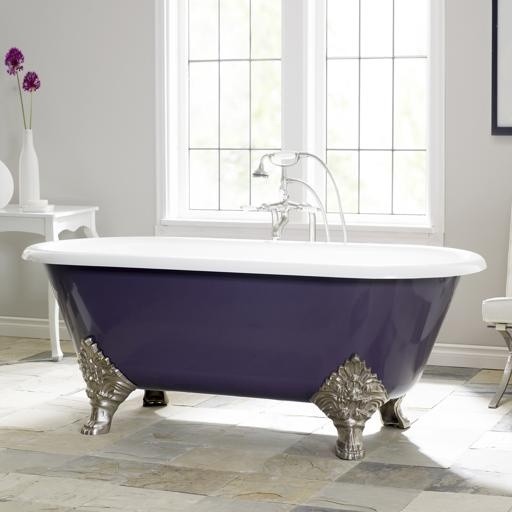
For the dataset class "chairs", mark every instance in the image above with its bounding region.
[482,224,512,408]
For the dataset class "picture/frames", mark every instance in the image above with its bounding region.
[492,0,512,135]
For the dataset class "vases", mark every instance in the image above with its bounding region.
[18,129,39,208]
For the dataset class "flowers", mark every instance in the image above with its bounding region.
[4,47,41,129]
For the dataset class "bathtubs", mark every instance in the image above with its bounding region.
[21,236,487,461]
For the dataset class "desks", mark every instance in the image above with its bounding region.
[0,203,100,362]
